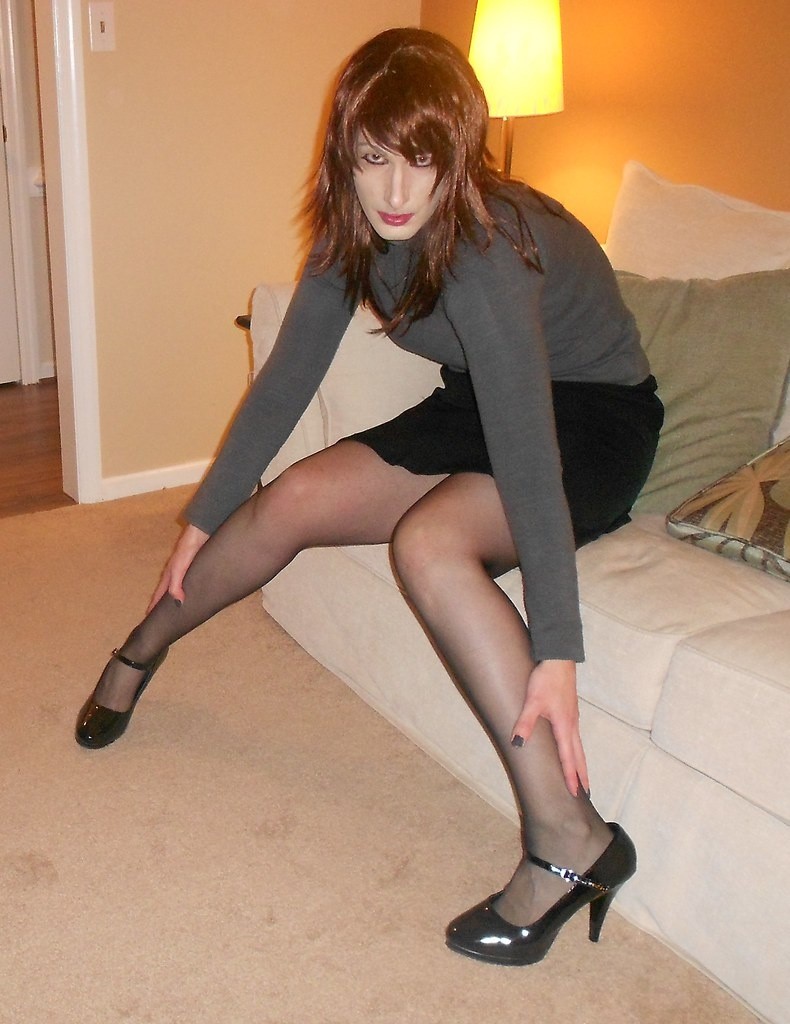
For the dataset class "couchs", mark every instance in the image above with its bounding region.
[247,242,790,1024]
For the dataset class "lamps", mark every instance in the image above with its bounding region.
[468,0,563,181]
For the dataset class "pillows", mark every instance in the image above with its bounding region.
[613,269,790,518]
[606,159,790,451]
[663,435,790,583]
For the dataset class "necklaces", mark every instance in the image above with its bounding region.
[372,236,419,318]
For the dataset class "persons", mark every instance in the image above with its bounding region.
[72,28,667,968]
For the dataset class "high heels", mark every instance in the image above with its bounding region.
[74,636,169,750]
[445,822,637,967]
